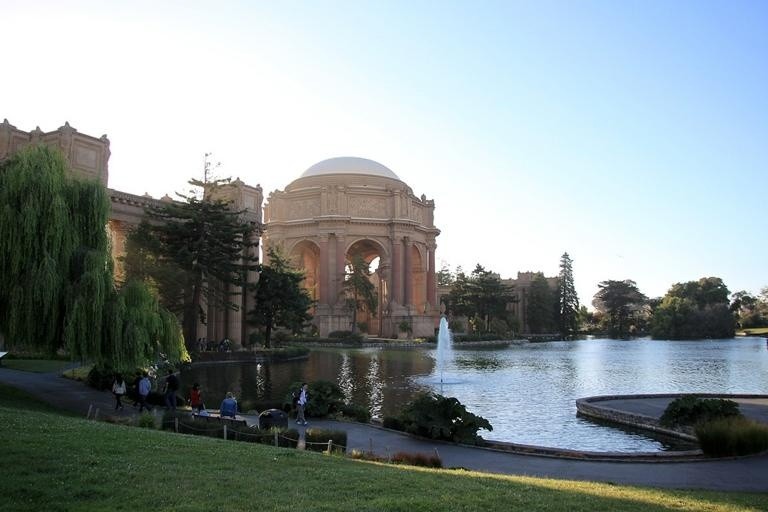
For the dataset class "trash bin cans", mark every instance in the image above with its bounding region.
[258,409,289,430]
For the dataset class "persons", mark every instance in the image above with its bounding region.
[291,382,309,425]
[112,372,126,411]
[139,370,153,412]
[132,371,143,407]
[220,391,240,420]
[163,368,178,411]
[197,336,231,353]
[191,381,206,416]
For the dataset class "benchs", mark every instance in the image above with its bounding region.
[192,413,247,430]
